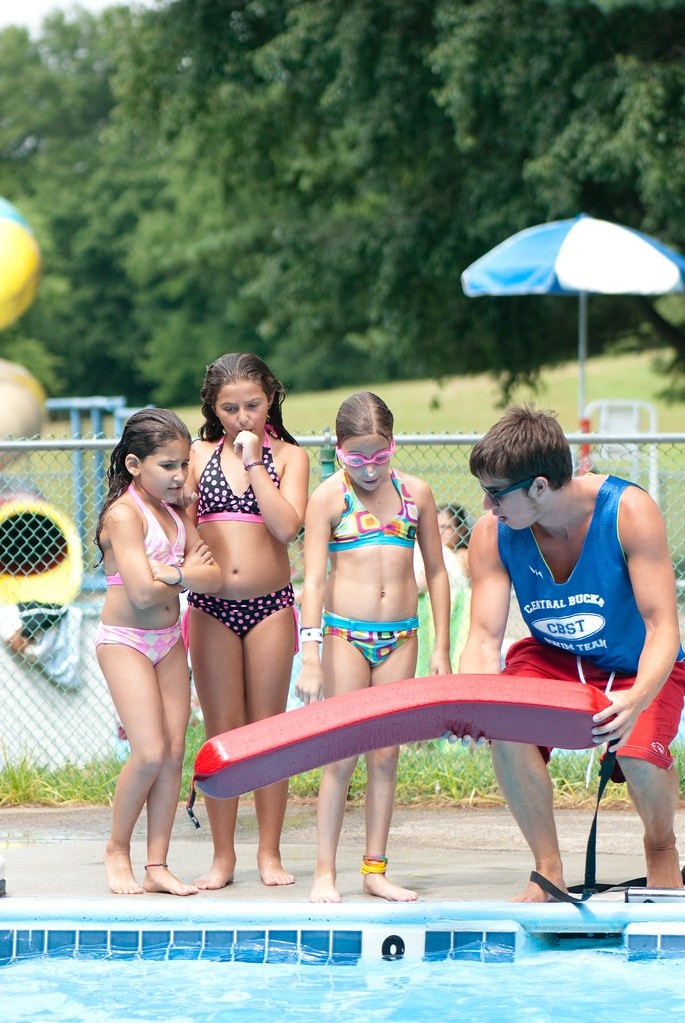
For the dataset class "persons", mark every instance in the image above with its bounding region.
[93,408,224,896]
[455,408,685,903]
[413,503,471,594]
[295,392,453,903]
[174,352,309,890]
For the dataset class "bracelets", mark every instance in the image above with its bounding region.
[300,627,323,642]
[171,564,182,584]
[245,461,264,471]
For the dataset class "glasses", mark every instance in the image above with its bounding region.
[480,476,535,507]
[336,437,396,468]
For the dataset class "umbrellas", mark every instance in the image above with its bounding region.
[459,217,685,420]
